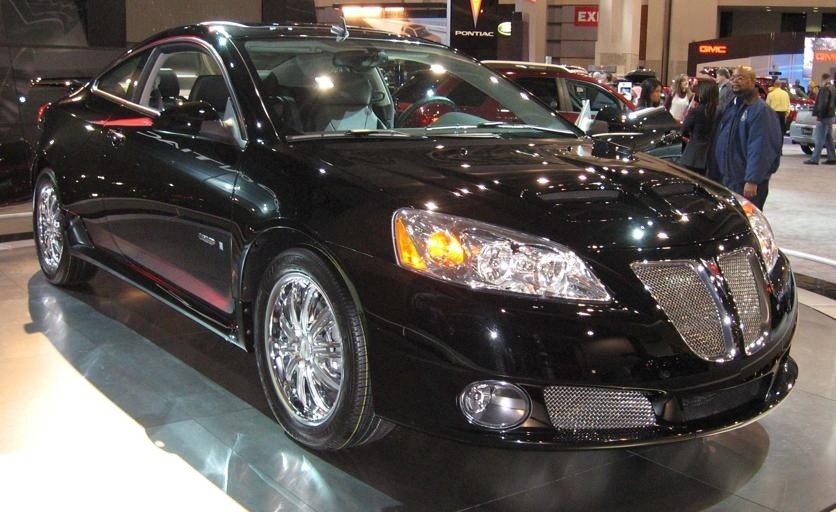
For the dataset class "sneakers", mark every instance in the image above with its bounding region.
[821,158,836,164]
[803,159,818,164]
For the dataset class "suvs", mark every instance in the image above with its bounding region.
[389,60,688,145]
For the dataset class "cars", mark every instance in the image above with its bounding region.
[790,105,836,154]
[755,78,815,130]
[31,20,797,451]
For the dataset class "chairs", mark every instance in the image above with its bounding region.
[152,68,381,148]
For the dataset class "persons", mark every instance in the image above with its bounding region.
[588,65,836,211]
